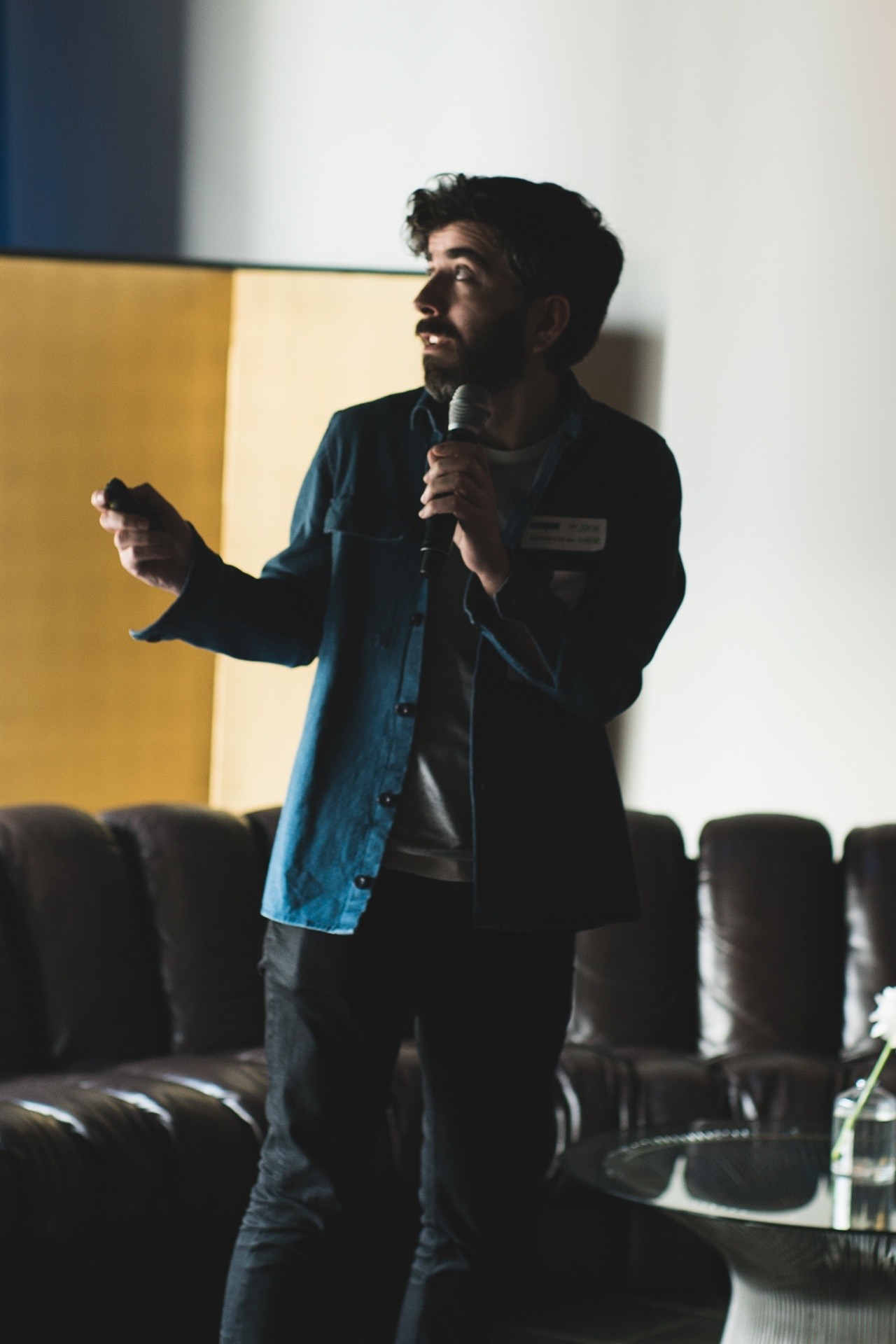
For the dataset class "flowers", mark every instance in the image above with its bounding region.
[826,982,896,1156]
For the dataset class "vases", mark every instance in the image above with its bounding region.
[829,1075,896,1184]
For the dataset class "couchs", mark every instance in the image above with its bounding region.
[0,799,896,1341]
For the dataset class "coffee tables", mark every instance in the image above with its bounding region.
[558,1120,895,1344]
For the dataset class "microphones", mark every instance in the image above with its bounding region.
[418,384,494,575]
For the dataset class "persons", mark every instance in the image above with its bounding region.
[84,175,691,1344]
